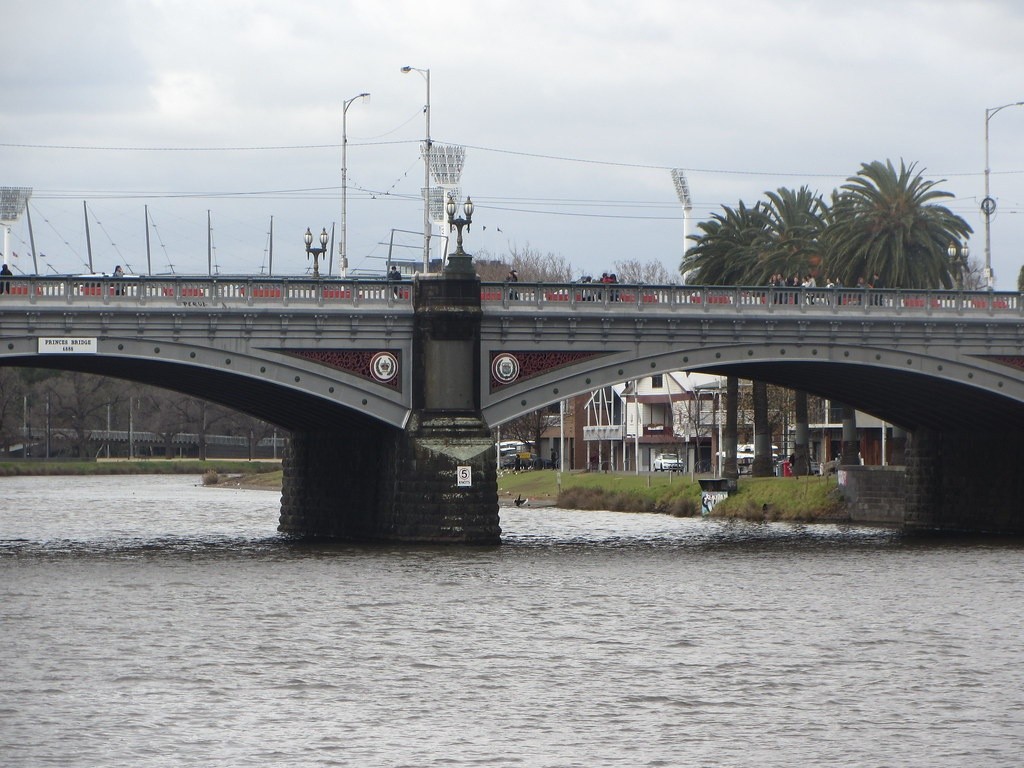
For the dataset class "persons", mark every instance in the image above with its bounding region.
[512,448,556,474]
[789,453,795,471]
[506,270,519,300]
[388,266,401,299]
[113,266,125,296]
[581,273,619,301]
[0,264,12,295]
[768,273,883,306]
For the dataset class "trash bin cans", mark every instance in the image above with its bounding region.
[775,461,782,477]
[783,461,791,477]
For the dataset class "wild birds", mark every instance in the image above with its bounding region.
[512,493,528,507]
[495,465,534,494]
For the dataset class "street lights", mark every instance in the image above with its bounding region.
[947,240,971,287]
[983,101,1024,286]
[302,227,331,279]
[445,192,474,256]
[401,64,431,272]
[339,91,372,274]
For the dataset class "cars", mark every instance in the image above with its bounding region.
[653,453,684,472]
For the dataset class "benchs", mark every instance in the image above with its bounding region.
[324,289,351,300]
[545,291,581,301]
[972,296,1010,309]
[399,290,410,299]
[902,295,941,308]
[621,293,658,303]
[761,295,794,304]
[692,294,731,304]
[241,287,280,297]
[10,287,41,296]
[840,296,859,304]
[164,288,205,307]
[482,292,501,300]
[81,287,115,295]
